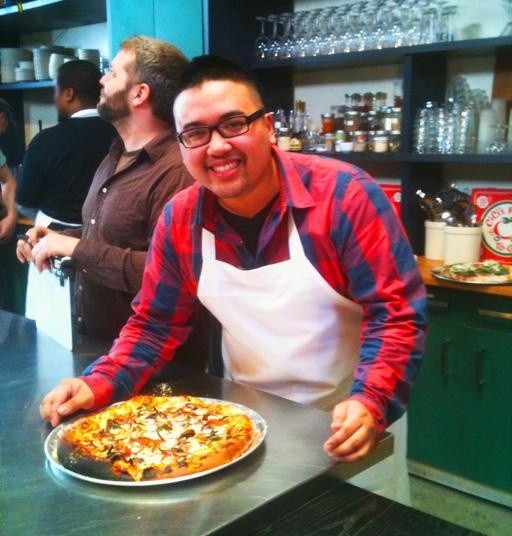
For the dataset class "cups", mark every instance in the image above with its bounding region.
[412,76,512,155]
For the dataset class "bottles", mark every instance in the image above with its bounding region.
[416,183,480,228]
[270,92,402,154]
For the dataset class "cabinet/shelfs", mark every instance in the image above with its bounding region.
[0,0,108,170]
[202,0,512,259]
[407,285,512,495]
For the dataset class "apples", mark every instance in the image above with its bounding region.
[495,217,512,238]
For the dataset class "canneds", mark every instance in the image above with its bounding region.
[277,128,291,152]
[321,103,401,153]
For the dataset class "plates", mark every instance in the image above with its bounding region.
[0,46,100,83]
[431,264,512,285]
[35,441,268,504]
[44,396,269,487]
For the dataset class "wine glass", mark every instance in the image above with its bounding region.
[499,0,512,38]
[255,1,456,60]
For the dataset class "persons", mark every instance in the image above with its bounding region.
[1,98,26,168]
[35,51,432,509]
[0,150,18,246]
[14,33,205,368]
[13,57,120,320]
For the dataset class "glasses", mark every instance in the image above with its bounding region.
[175,107,266,150]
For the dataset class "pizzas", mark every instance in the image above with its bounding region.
[432,260,512,284]
[61,396,253,483]
